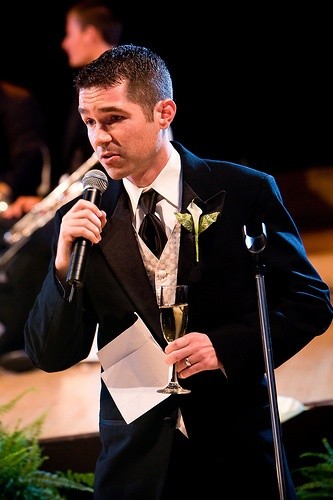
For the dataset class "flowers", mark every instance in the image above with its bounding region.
[173,200,220,262]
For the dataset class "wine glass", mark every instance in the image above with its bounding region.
[156,285,192,394]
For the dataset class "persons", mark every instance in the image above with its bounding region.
[21,44,332,499]
[0,1,142,223]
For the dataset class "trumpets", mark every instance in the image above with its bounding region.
[0,153,99,282]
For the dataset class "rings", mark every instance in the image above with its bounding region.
[183,357,191,368]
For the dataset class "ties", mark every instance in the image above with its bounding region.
[138,190,167,260]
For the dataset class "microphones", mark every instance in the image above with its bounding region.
[66,169,108,302]
